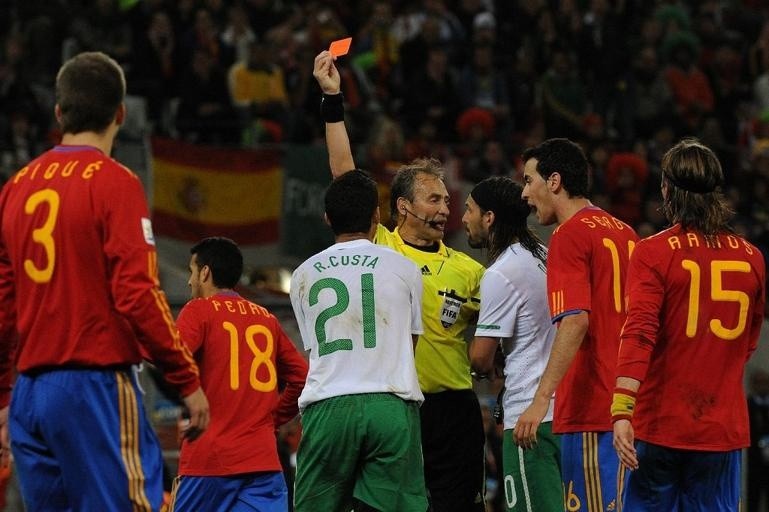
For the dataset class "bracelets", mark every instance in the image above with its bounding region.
[610,388,637,422]
[321,92,345,122]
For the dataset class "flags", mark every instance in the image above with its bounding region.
[144,133,283,242]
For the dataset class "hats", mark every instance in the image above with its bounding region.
[471,177,531,221]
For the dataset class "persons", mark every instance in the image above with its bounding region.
[462,176,564,511]
[168,237,308,511]
[0,1,769,245]
[612,139,766,511]
[0,52,210,511]
[289,169,428,511]
[521,137,641,511]
[313,50,486,511]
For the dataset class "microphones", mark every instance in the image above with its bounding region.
[406,209,436,227]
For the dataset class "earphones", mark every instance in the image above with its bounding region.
[401,205,407,209]
